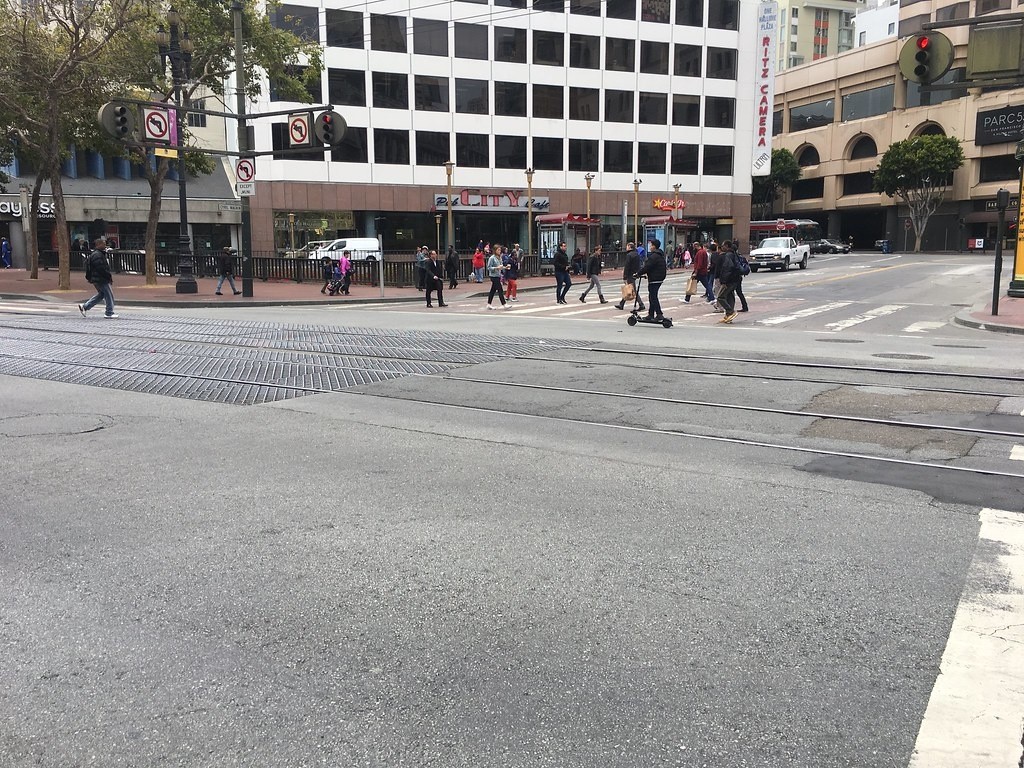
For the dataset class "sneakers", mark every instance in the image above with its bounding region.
[679,299,689,304]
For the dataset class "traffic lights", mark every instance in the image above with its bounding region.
[315,111,347,146]
[899,31,955,85]
[97,100,136,140]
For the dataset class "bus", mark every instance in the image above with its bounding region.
[750,219,822,254]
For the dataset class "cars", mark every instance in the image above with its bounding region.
[821,238,851,254]
[283,240,335,265]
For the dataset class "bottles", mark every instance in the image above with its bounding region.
[471,272,474,278]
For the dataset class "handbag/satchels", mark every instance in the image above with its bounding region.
[621,284,636,301]
[686,278,697,295]
[348,269,355,275]
[740,263,750,275]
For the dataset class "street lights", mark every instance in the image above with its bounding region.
[673,183,682,220]
[442,160,456,246]
[584,173,595,253]
[434,215,443,260]
[524,167,535,255]
[286,212,296,251]
[633,178,642,247]
[153,4,199,294]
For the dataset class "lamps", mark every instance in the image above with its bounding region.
[217,211,221,216]
[83,209,89,213]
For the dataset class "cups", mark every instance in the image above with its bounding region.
[500,265,503,269]
[507,264,511,270]
[601,262,605,267]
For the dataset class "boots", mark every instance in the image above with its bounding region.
[637,301,645,311]
[599,295,608,303]
[579,293,586,303]
[615,299,626,310]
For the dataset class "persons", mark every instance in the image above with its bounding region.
[321,260,333,294]
[338,250,350,295]
[609,240,621,269]
[71,237,87,251]
[487,245,512,311]
[333,261,341,280]
[1,237,11,269]
[443,245,459,289]
[484,242,491,256]
[553,242,572,304]
[579,246,608,304]
[632,240,666,320]
[78,238,118,317]
[110,239,116,248]
[666,239,749,322]
[478,240,484,252]
[416,246,429,291]
[426,250,449,307]
[473,248,484,283]
[215,247,243,295]
[615,242,646,311]
[500,244,524,301]
[571,247,587,274]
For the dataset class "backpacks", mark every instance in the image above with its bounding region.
[5,242,11,250]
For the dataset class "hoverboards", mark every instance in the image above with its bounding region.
[627,275,673,328]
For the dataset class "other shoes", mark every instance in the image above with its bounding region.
[215,292,223,295]
[504,305,512,311]
[78,304,86,317]
[427,304,433,308]
[321,290,326,294]
[700,294,748,323]
[104,314,119,318]
[505,298,519,302]
[557,299,567,304]
[642,313,664,321]
[439,302,448,307]
[486,304,496,310]
[234,291,242,295]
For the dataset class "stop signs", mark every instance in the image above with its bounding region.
[777,221,785,230]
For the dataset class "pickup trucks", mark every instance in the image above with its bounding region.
[748,237,811,273]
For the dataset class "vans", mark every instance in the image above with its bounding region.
[308,238,381,269]
